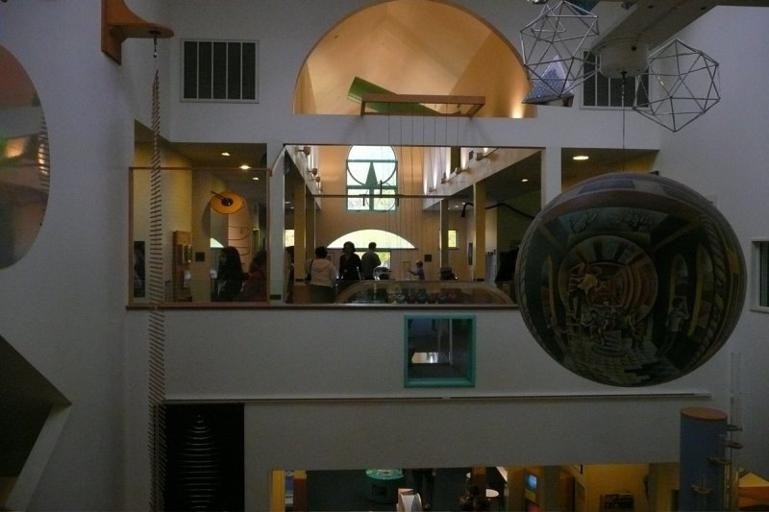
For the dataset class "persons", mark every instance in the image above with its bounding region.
[339,240,361,282]
[212,246,244,300]
[285,245,310,303]
[653,297,691,359]
[244,254,267,300]
[306,246,337,303]
[359,241,381,279]
[411,468,440,510]
[568,266,603,324]
[408,260,425,280]
[256,251,267,269]
